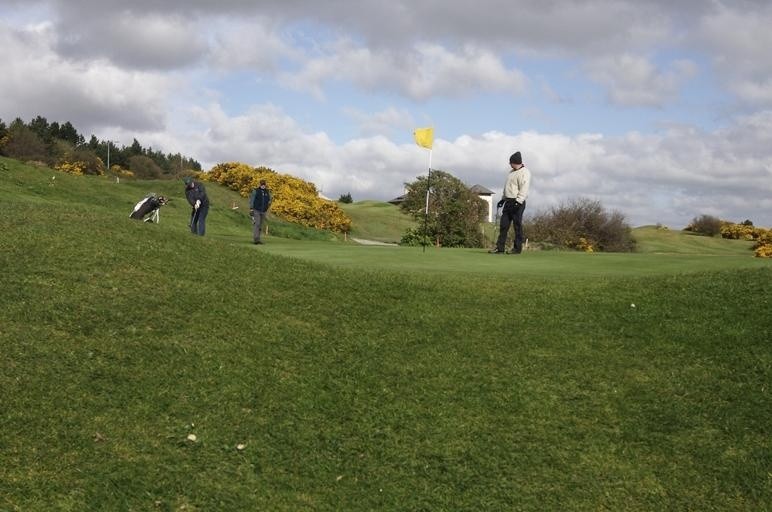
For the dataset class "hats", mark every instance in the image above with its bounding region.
[260,180,265,185]
[510,152,522,164]
[184,177,192,184]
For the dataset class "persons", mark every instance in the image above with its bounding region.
[487,151,532,254]
[184,177,210,237]
[248,179,272,246]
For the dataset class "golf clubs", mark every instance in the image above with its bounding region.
[188,206,198,228]
[251,215,256,225]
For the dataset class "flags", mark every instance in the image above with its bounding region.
[414,127,434,150]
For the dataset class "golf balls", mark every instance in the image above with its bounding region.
[630,303,636,308]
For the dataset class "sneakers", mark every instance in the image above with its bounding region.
[489,247,504,254]
[255,241,262,245]
[506,249,520,254]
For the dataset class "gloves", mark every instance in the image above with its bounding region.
[249,209,254,216]
[194,200,201,210]
[513,201,521,208]
[497,199,505,208]
